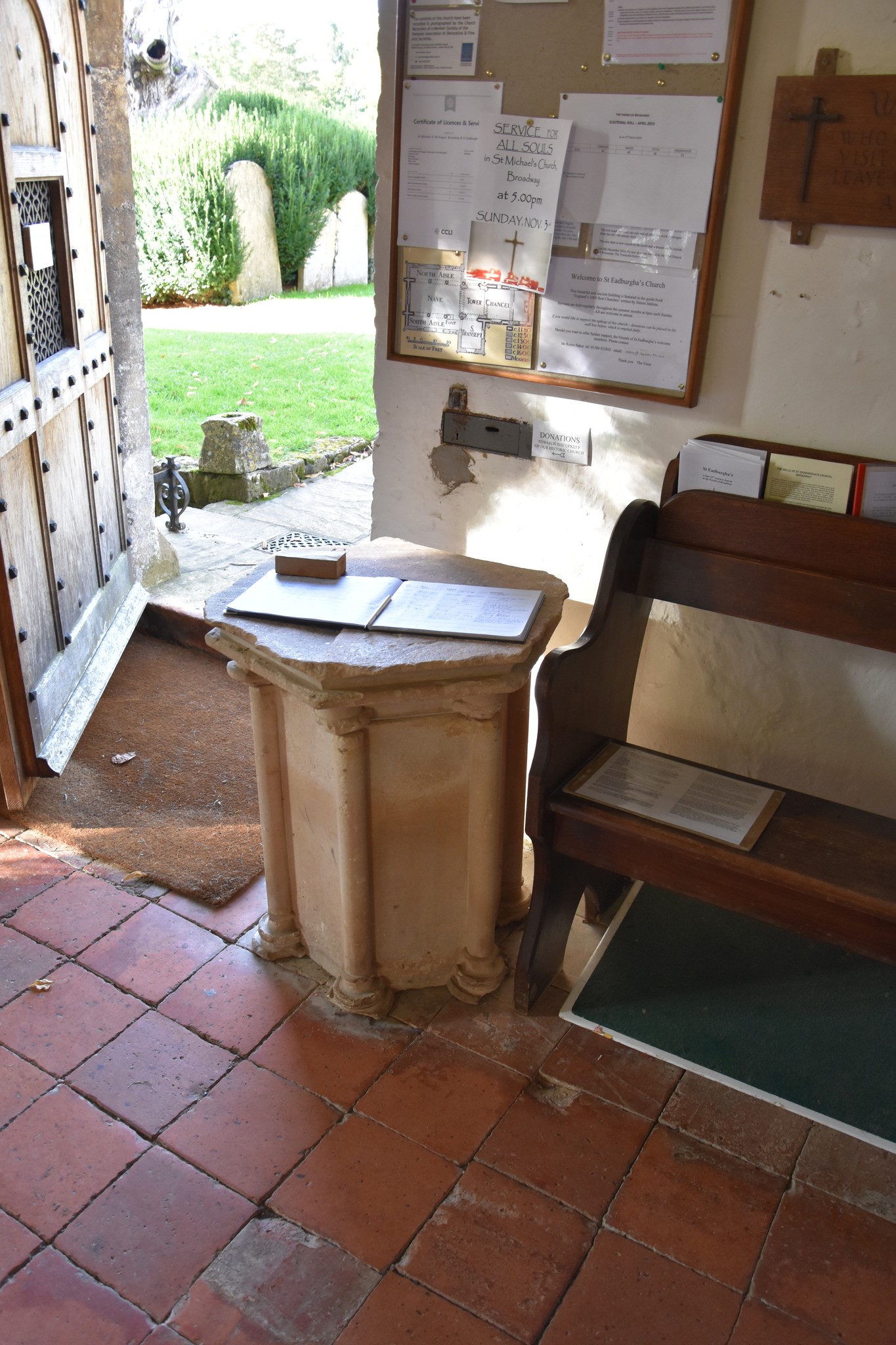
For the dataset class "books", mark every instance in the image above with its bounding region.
[223,569,546,644]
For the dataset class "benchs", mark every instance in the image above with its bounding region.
[517,494,896,1031]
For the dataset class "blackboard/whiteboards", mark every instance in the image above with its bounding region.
[559,881,896,1157]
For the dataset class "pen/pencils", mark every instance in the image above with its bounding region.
[363,594,392,629]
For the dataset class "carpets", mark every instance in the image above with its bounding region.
[556,876,896,1155]
